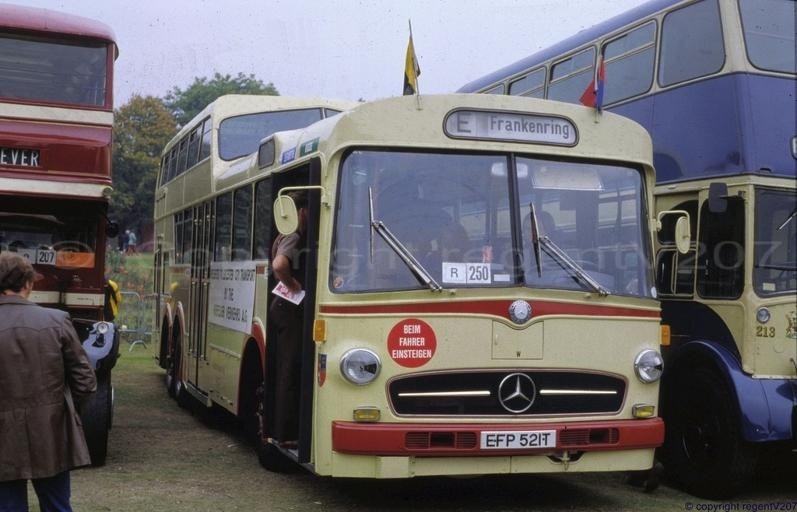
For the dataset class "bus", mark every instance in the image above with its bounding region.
[149,83,674,480]
[441,2,797,499]
[0,1,119,468]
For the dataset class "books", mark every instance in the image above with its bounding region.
[272,281,306,306]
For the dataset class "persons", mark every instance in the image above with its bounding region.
[264,189,318,449]
[0,251,98,512]
[122,228,138,256]
